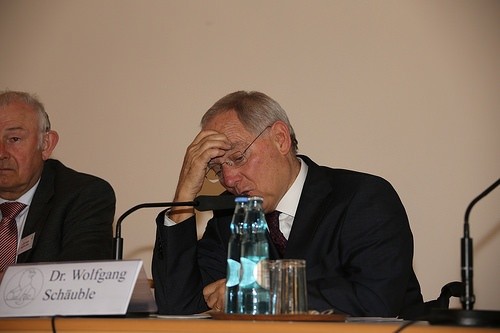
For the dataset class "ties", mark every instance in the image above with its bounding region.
[265,210,288,259]
[0,201,28,283]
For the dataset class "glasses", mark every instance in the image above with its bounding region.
[204,125,272,184]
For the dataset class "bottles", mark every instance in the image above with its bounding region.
[225,197,248,315]
[238,197,269,314]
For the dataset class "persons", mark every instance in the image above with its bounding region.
[151,91,424,318]
[0,90,116,283]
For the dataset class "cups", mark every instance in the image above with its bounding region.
[267,260,282,313]
[276,258,308,315]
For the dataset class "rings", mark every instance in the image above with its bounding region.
[212,304,222,313]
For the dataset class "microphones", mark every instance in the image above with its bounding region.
[113,195,250,260]
[430,177,500,328]
